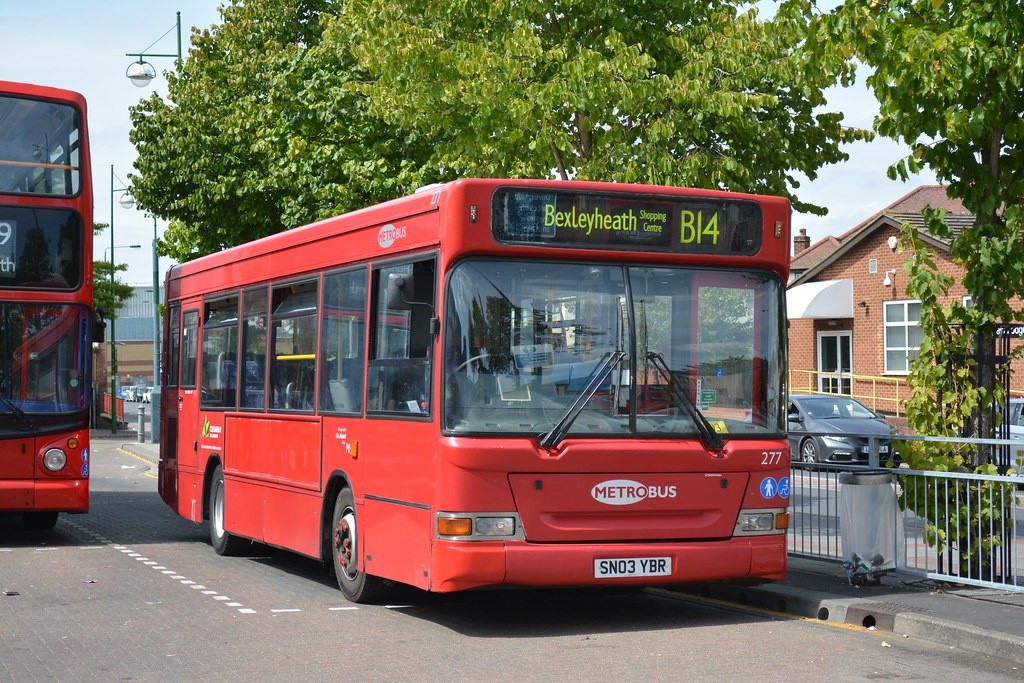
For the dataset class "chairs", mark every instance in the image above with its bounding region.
[286,379,322,410]
[201,386,216,407]
[244,382,284,409]
[821,404,835,416]
[217,352,262,401]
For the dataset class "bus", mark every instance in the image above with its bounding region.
[155,177,787,603]
[0,81,105,534]
[252,310,410,364]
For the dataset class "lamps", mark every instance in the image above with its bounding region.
[858,301,869,308]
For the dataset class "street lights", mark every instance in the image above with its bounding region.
[108,164,138,434]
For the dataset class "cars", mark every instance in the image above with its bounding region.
[121,386,153,404]
[952,398,1024,487]
[744,395,907,476]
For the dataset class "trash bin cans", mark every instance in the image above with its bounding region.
[837,470,902,585]
